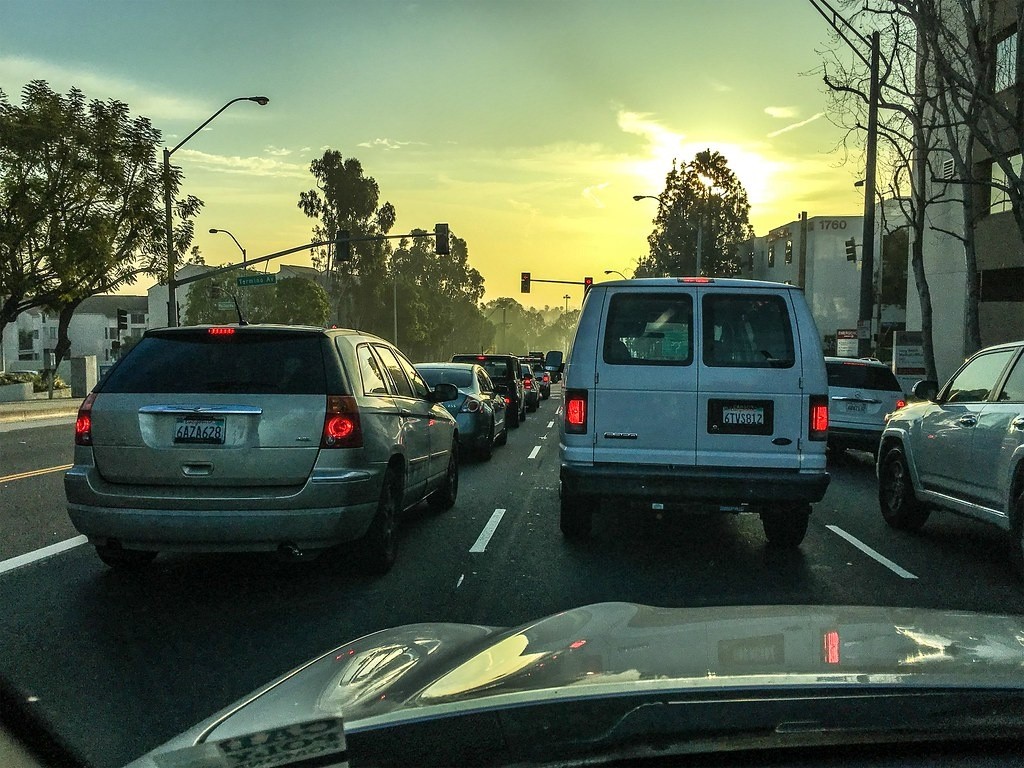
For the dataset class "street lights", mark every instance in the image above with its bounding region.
[634,195,703,276]
[163,95,271,329]
[209,228,247,271]
[562,292,572,359]
[856,177,882,349]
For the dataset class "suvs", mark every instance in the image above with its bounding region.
[63,320,460,573]
[822,355,905,458]
[451,351,533,427]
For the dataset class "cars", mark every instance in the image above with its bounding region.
[515,349,565,401]
[874,341,1024,561]
[491,363,543,414]
[410,362,509,462]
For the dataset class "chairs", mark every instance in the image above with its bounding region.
[714,341,733,363]
[608,340,632,359]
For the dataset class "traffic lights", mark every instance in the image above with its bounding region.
[584,277,593,293]
[845,237,857,264]
[116,307,127,330]
[521,272,530,293]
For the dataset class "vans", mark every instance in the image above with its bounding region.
[544,276,832,551]
